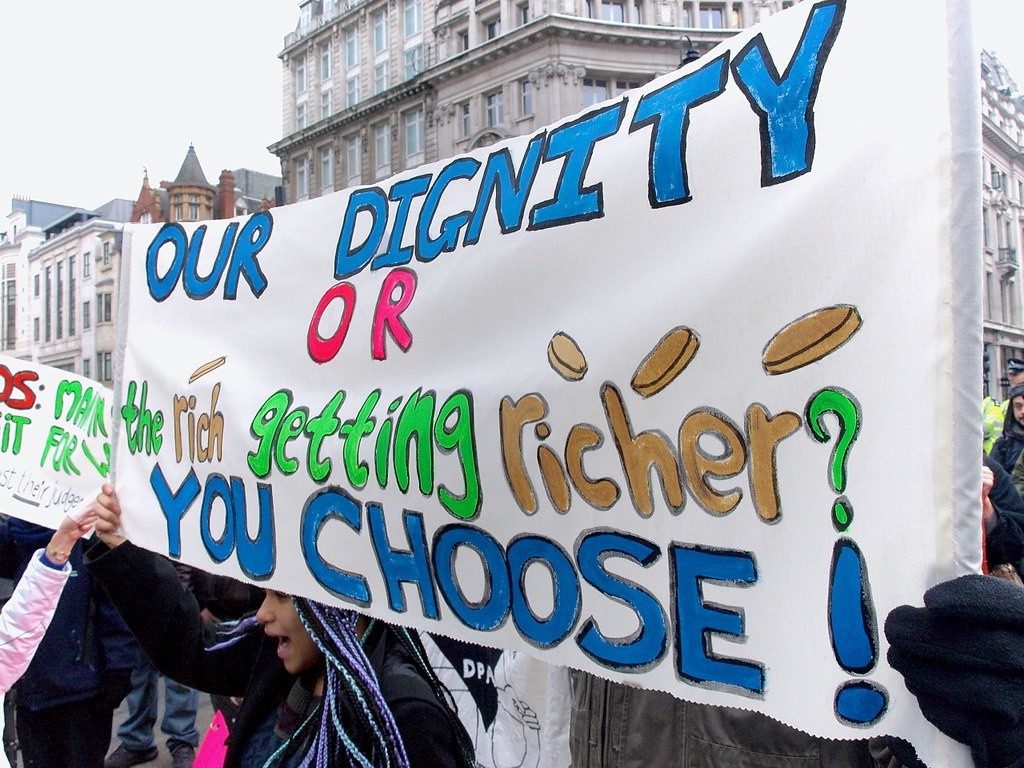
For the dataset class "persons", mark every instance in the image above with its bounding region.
[0,358,1024,768]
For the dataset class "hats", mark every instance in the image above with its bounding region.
[1006,358,1024,374]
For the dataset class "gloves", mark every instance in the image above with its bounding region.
[884,573,1024,768]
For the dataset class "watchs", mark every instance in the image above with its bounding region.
[47,543,70,562]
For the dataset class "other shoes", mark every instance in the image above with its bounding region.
[172,746,195,768]
[104,741,158,768]
